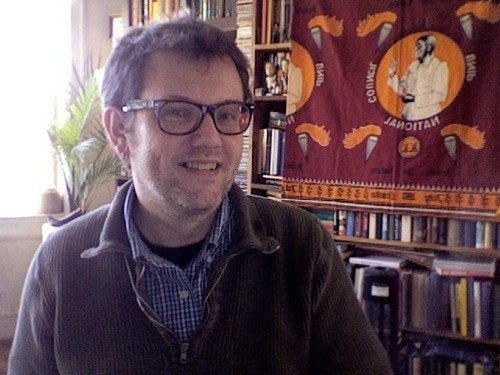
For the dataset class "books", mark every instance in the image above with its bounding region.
[130,1,238,29]
[262,190,500,252]
[372,325,500,375]
[253,0,294,188]
[334,239,500,343]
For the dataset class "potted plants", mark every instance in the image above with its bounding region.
[39,52,129,244]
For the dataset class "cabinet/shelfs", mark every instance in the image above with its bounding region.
[104,0,500,375]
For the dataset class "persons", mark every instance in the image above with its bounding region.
[5,12,394,374]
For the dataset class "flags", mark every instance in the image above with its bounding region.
[280,0,500,213]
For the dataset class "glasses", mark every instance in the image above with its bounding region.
[120,99,255,137]
